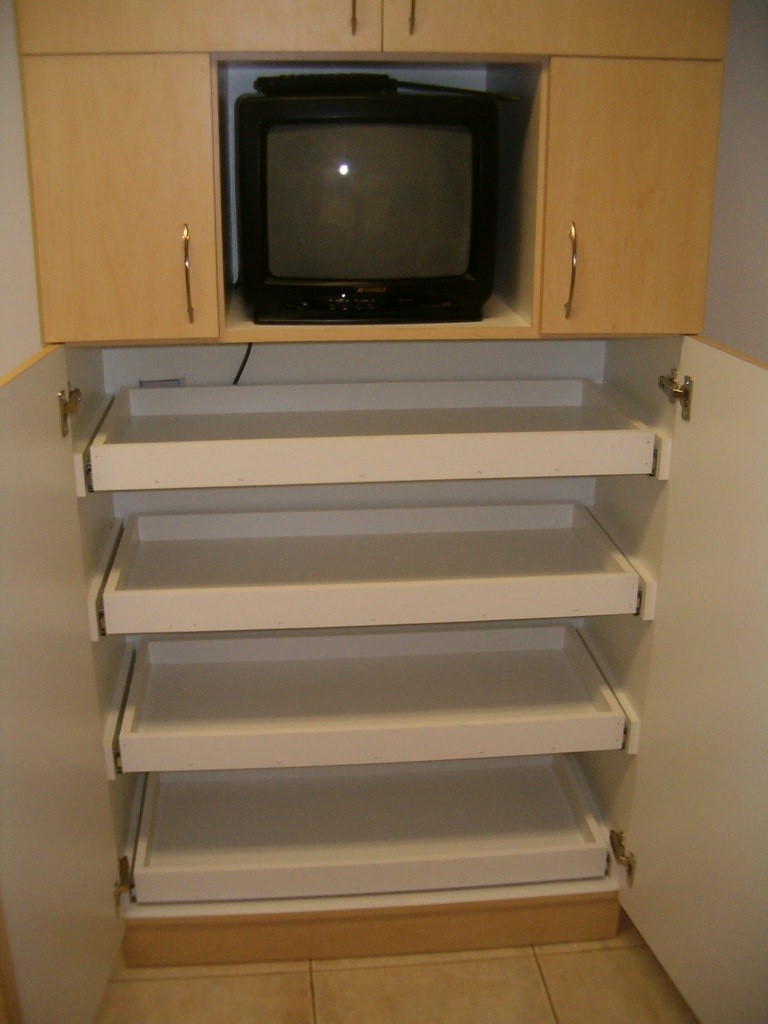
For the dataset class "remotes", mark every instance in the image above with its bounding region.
[254,73,394,96]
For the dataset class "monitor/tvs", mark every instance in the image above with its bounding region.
[235,91,502,323]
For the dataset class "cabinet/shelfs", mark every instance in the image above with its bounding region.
[0,0,768,1024]
